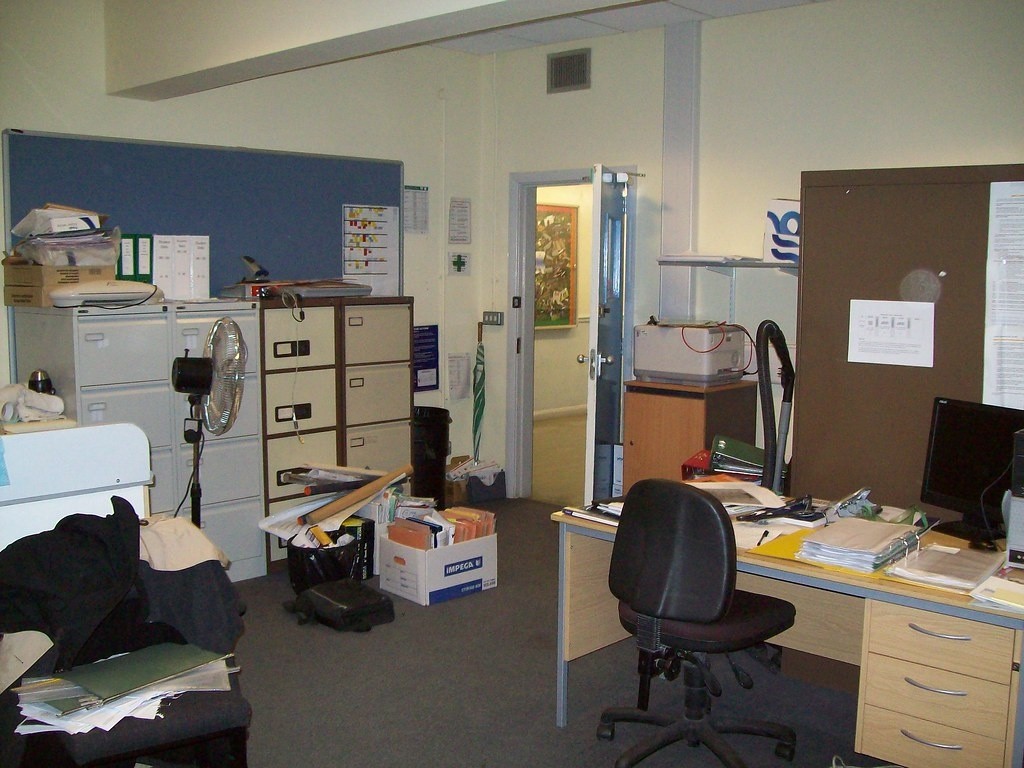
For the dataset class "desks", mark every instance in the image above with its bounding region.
[548,496,1024,768]
[0,423,155,553]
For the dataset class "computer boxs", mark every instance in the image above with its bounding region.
[1005,428,1024,570]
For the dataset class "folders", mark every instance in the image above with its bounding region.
[590,496,626,520]
[681,434,787,486]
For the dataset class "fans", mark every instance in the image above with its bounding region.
[172,316,247,616]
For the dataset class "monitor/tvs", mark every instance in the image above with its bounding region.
[920,397,1024,543]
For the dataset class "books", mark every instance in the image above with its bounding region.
[786,506,826,527]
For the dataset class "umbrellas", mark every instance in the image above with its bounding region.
[472,321,485,466]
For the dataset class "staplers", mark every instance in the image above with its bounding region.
[238,255,270,284]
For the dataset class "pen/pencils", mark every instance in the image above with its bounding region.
[756,529,769,546]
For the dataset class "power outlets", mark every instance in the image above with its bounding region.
[483,312,501,325]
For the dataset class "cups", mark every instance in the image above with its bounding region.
[28,369,52,394]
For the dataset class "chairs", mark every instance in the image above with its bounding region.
[0,496,251,768]
[596,479,798,768]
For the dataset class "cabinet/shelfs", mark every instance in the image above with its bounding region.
[13,302,267,583]
[261,296,413,573]
[622,380,759,497]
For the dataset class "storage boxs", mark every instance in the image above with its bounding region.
[763,198,800,263]
[36,216,100,233]
[114,233,210,301]
[4,264,115,308]
[444,456,470,507]
[340,514,498,606]
[305,525,333,548]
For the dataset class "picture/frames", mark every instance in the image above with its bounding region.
[534,203,579,330]
